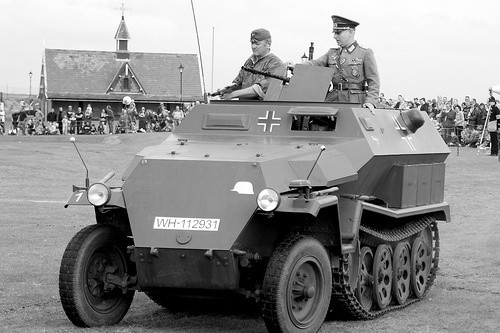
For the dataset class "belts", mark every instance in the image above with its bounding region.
[332,82,364,91]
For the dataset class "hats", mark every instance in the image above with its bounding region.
[332,15,359,32]
[251,29,270,42]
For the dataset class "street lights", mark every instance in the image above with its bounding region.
[29,71,33,98]
[178,62,184,107]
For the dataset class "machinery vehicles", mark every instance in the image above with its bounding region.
[56,62,455,333]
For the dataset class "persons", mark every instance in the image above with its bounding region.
[99,106,114,135]
[217,28,286,100]
[380,93,500,156]
[9,101,97,135]
[296,15,380,115]
[115,102,200,134]
